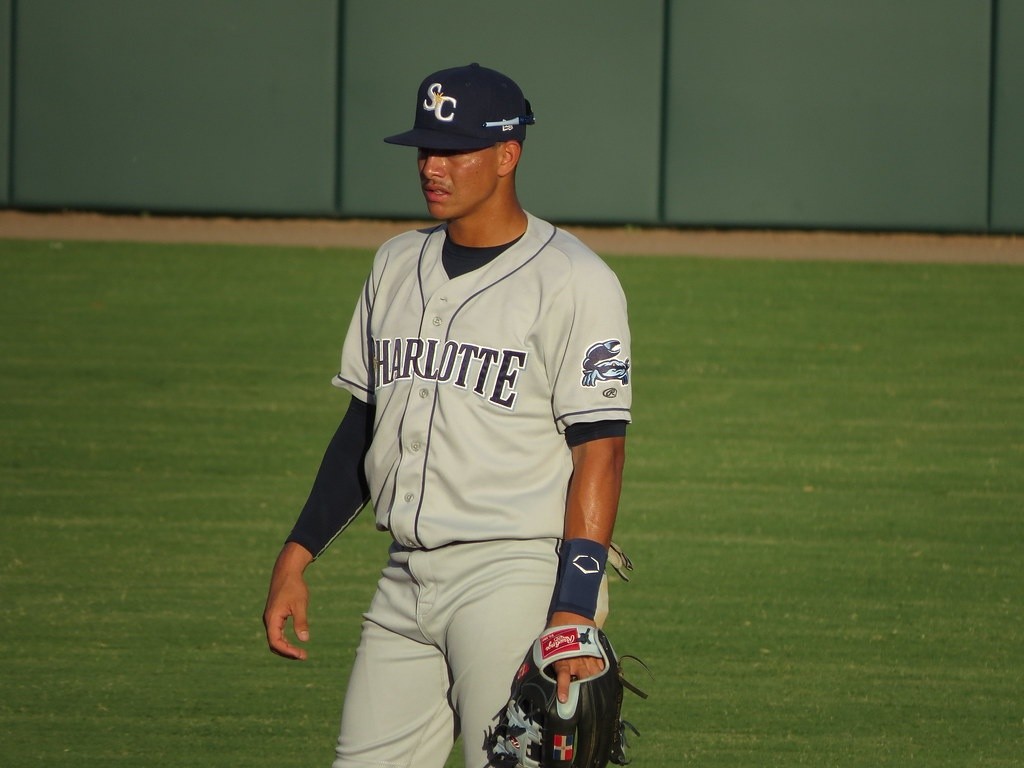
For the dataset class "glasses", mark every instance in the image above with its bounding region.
[482,99,536,128]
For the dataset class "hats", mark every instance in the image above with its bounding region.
[384,62,526,150]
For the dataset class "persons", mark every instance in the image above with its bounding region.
[263,63,648,768]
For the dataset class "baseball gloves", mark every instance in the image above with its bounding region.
[482,624,656,768]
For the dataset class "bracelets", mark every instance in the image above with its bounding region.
[552,538,608,621]
